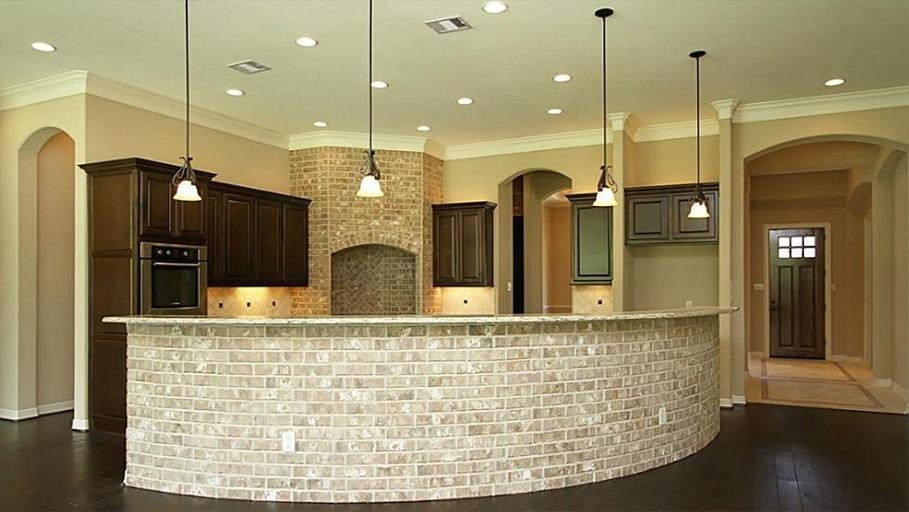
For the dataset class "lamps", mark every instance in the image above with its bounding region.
[356,176,384,199]
[481,0,509,15]
[545,107,564,116]
[224,88,245,97]
[686,202,710,218]
[371,80,389,89]
[416,124,432,132]
[172,180,202,202]
[294,36,318,48]
[29,40,57,54]
[312,121,329,128]
[591,188,618,207]
[552,72,573,84]
[456,96,474,106]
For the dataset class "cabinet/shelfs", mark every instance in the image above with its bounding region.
[282,193,312,287]
[223,182,254,288]
[77,157,218,243]
[431,200,497,287]
[254,188,282,288]
[207,179,223,288]
[564,192,612,285]
[623,181,719,246]
[102,305,741,502]
